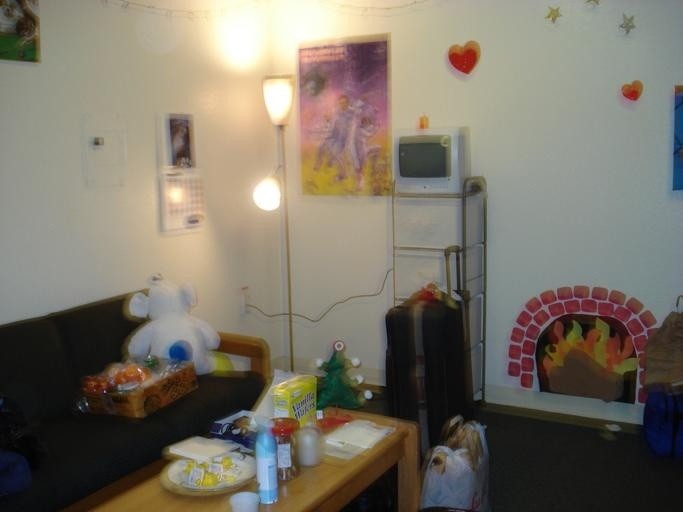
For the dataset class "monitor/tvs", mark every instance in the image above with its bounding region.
[392,127,463,196]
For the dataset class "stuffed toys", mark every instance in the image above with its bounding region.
[125,274,222,376]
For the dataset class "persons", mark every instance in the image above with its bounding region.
[172,120,187,165]
[347,87,384,195]
[312,93,353,182]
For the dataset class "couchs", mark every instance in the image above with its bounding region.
[0,290,270,512]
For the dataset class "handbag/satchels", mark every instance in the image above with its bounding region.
[643,391,683,458]
[421,414,489,512]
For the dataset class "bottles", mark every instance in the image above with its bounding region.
[254,414,279,504]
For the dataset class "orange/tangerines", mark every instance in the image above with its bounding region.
[86,366,151,392]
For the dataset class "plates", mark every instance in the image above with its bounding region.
[166,451,256,491]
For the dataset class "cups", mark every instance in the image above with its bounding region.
[228,491,260,512]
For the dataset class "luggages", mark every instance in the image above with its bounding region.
[386,246,470,465]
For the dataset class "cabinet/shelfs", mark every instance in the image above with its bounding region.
[391,176,487,411]
